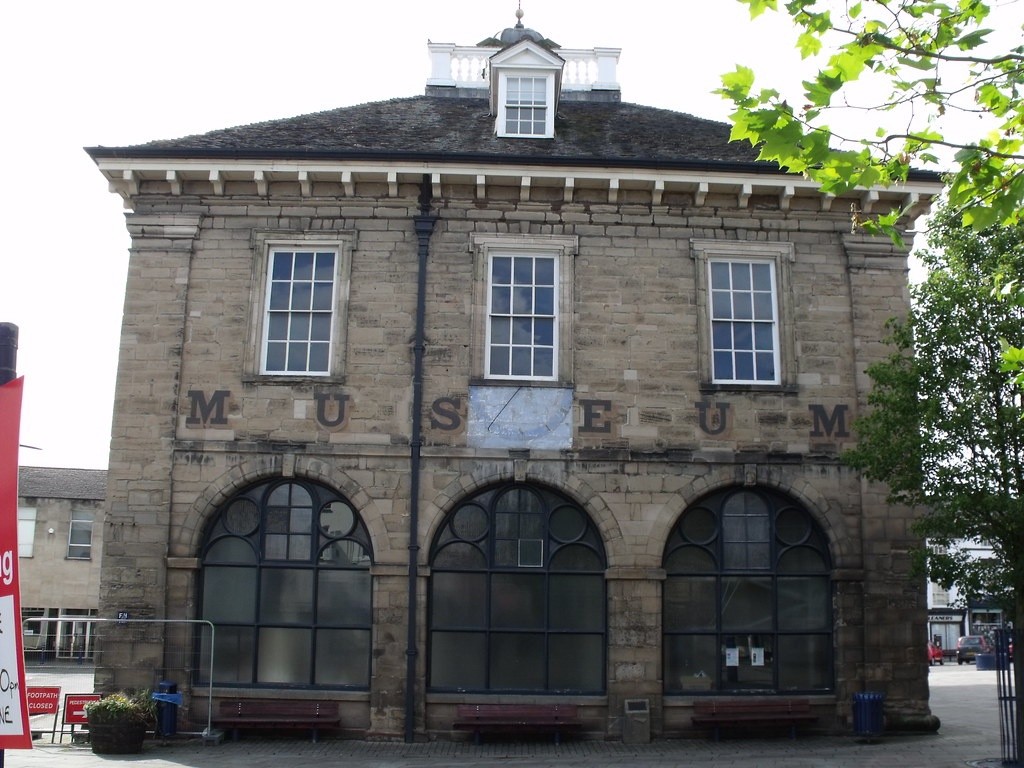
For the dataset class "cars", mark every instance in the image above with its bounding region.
[955,635,991,665]
[927,642,944,666]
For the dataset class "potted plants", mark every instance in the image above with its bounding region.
[83,691,148,755]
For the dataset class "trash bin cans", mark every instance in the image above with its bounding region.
[152,691,180,737]
[854,691,883,738]
[624,699,650,745]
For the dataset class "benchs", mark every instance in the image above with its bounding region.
[691,700,819,741]
[454,705,583,746]
[210,699,341,743]
